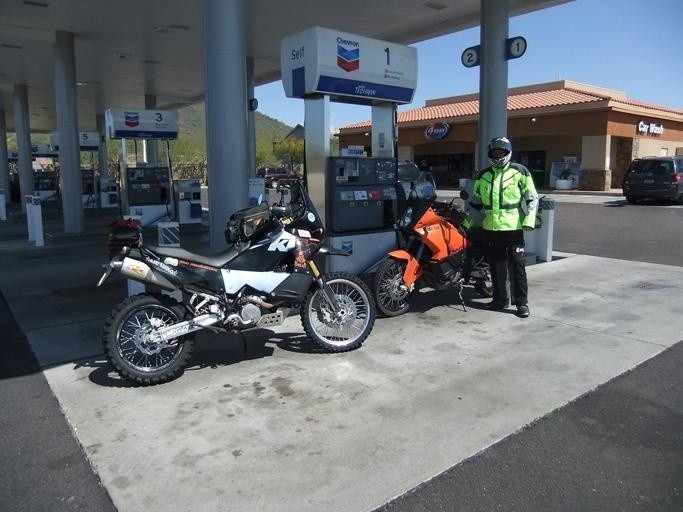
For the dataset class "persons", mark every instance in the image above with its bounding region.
[459,137,539,317]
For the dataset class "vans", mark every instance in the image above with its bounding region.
[396,161,437,202]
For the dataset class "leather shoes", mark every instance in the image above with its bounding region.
[516,304,531,318]
[485,299,510,311]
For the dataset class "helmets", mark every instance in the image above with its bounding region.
[487,135,513,170]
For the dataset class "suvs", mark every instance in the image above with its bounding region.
[257,167,305,188]
[622,155,683,205]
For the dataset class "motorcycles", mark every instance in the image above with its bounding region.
[375,180,494,317]
[96,183,377,384]
